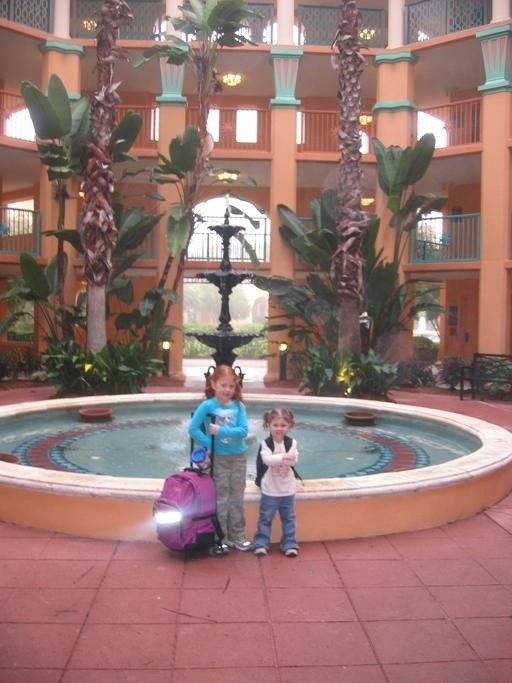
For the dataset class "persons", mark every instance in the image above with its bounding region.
[252,408,299,556]
[188,364,252,553]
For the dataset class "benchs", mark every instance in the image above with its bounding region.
[458,352,512,400]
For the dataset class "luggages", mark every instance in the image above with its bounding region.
[151,412,217,557]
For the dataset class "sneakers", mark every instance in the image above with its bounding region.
[284,548,298,557]
[213,538,254,556]
[254,548,267,557]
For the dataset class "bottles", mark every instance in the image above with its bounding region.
[192,446,215,475]
